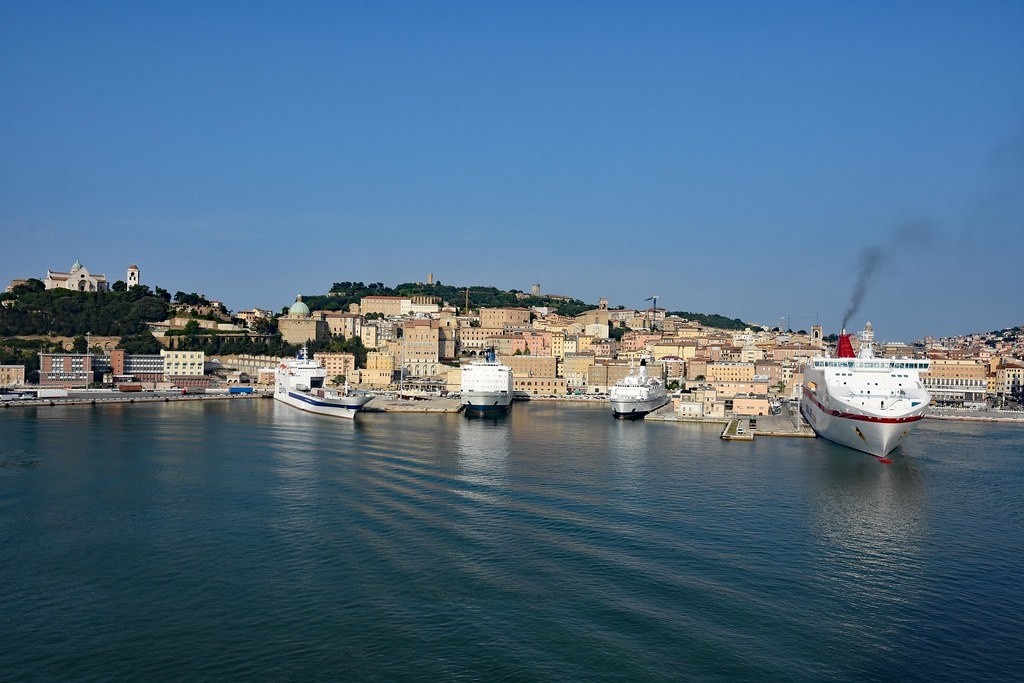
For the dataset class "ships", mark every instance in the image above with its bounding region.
[802,321,933,458]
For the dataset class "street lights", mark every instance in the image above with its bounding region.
[86,332,91,392]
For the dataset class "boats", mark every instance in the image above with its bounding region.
[609,356,672,419]
[458,362,514,419]
[512,391,531,402]
[272,341,375,420]
